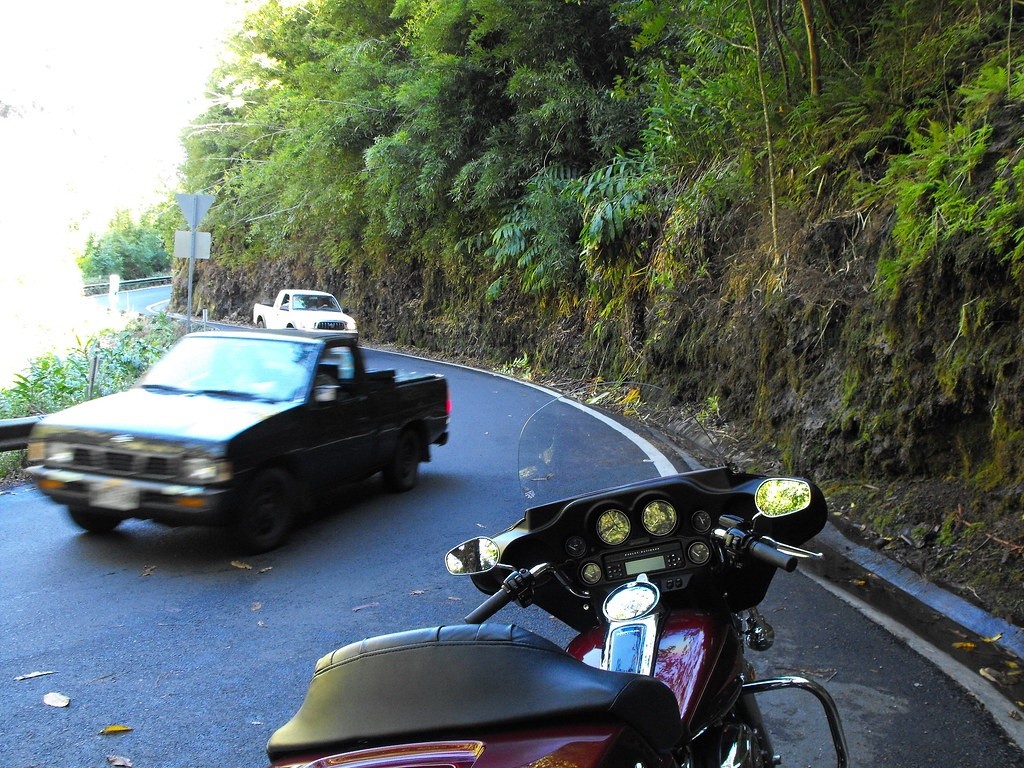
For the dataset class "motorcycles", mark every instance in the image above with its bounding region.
[269,463,849,768]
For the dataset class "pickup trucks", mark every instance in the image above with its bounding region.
[28,330,453,557]
[252,288,358,345]
